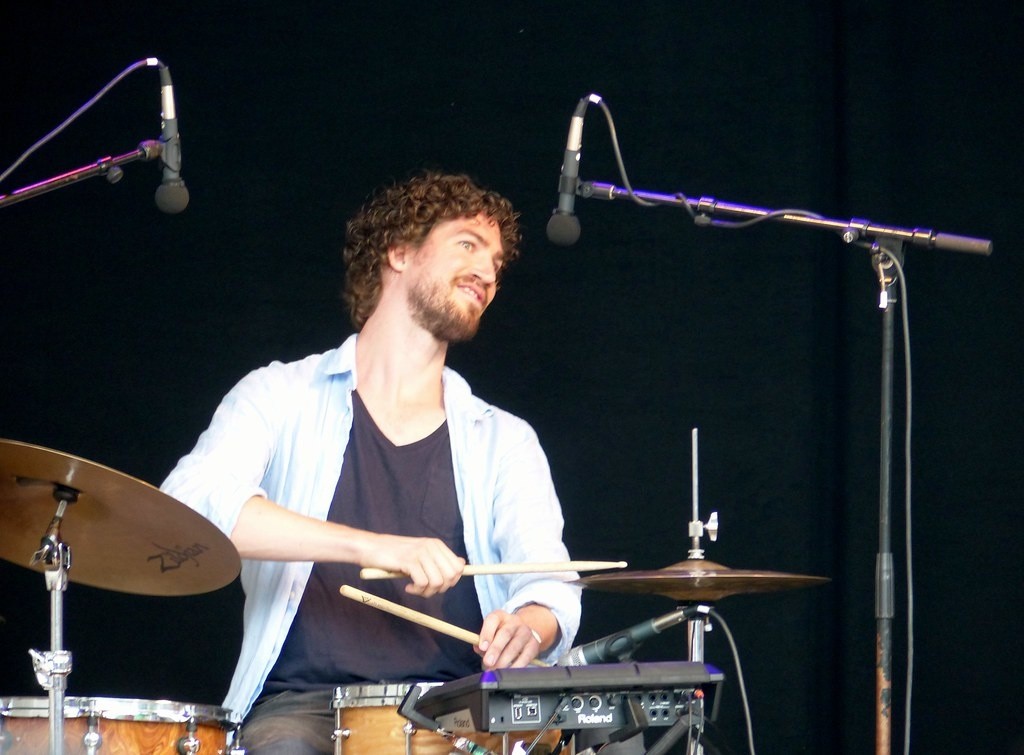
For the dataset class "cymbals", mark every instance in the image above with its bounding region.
[1,442,242,602]
[565,560,832,602]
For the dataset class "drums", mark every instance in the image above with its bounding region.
[0,695,232,754]
[330,680,570,755]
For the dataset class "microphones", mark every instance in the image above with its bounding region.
[567,604,712,666]
[547,93,601,245]
[147,58,190,213]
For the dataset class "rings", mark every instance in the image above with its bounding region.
[531,628,542,644]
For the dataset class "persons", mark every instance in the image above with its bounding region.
[158,174,581,755]
